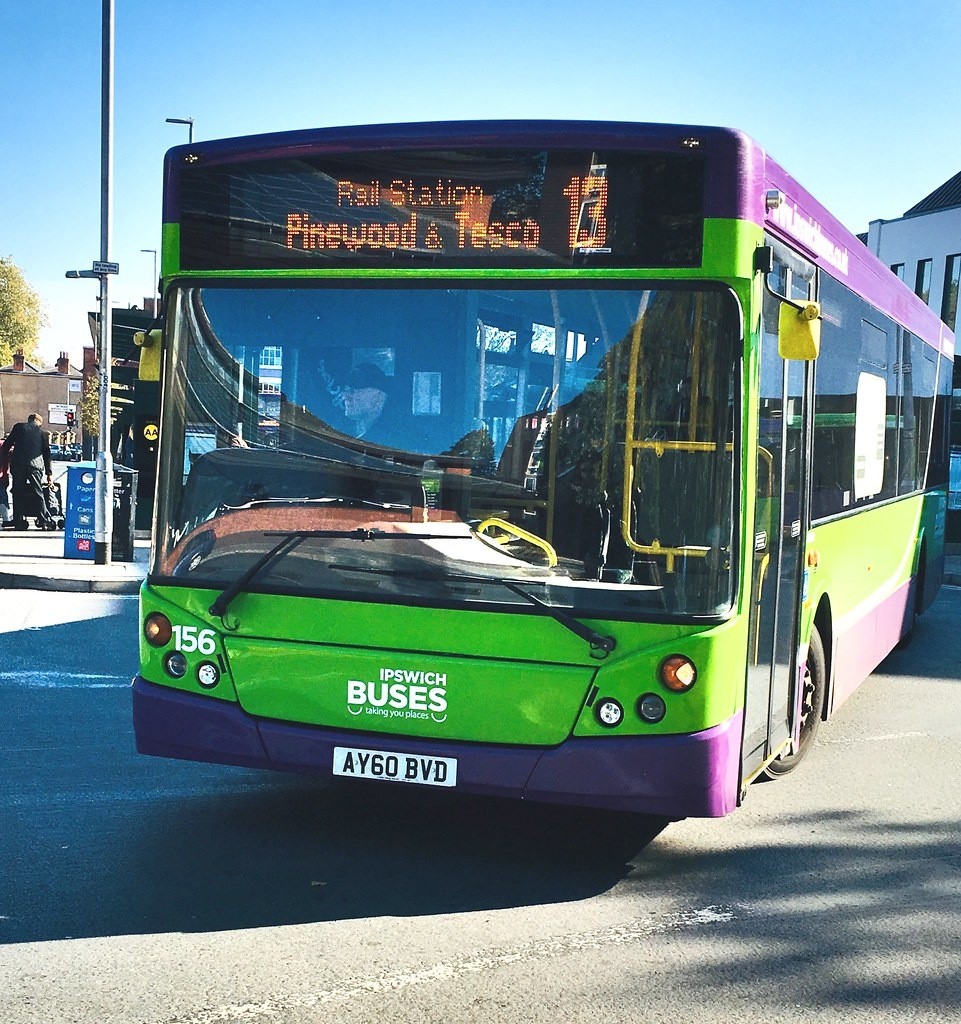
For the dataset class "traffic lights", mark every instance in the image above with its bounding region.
[67,412,74,427]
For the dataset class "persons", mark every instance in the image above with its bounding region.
[0,433,16,527]
[0,412,55,531]
[555,375,716,502]
[231,363,405,472]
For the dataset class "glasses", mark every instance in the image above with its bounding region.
[343,386,375,397]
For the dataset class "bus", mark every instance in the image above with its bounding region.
[129,116,954,829]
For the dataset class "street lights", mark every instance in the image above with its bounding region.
[140,249,159,320]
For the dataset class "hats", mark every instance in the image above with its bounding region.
[332,360,394,397]
[27,412,43,423]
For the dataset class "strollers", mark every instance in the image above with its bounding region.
[9,482,65,531]
